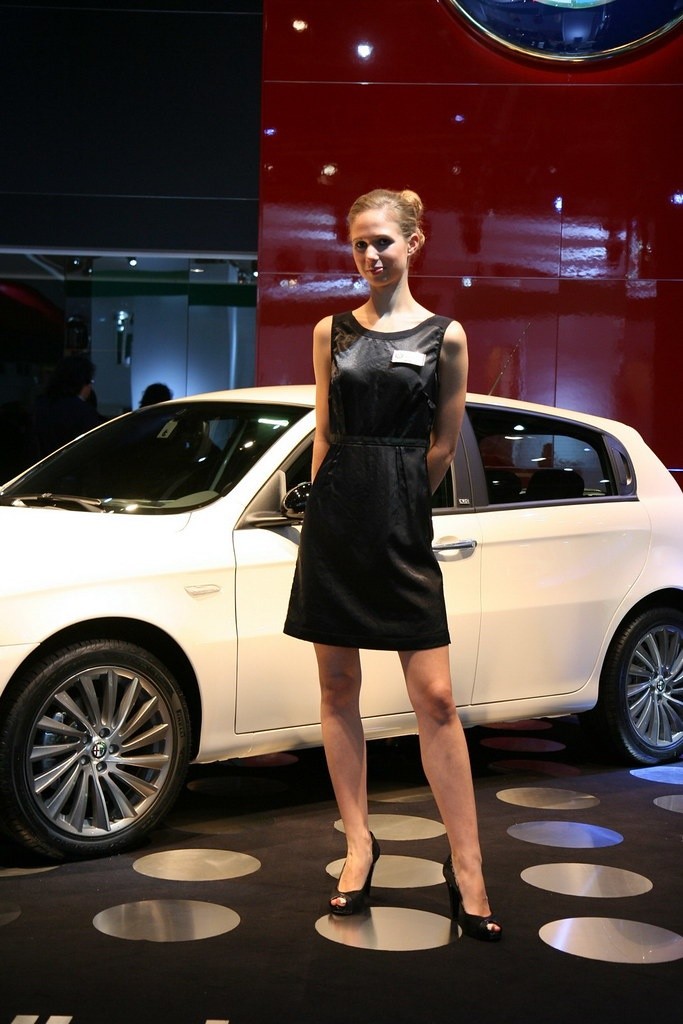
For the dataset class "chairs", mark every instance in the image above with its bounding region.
[517,469,588,502]
[205,421,275,493]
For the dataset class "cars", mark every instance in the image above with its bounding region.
[0,384,683,862]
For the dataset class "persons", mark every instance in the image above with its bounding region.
[283,188,507,946]
[37,356,111,480]
[114,384,174,484]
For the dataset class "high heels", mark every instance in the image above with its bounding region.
[328,830,381,920]
[440,854,504,943]
